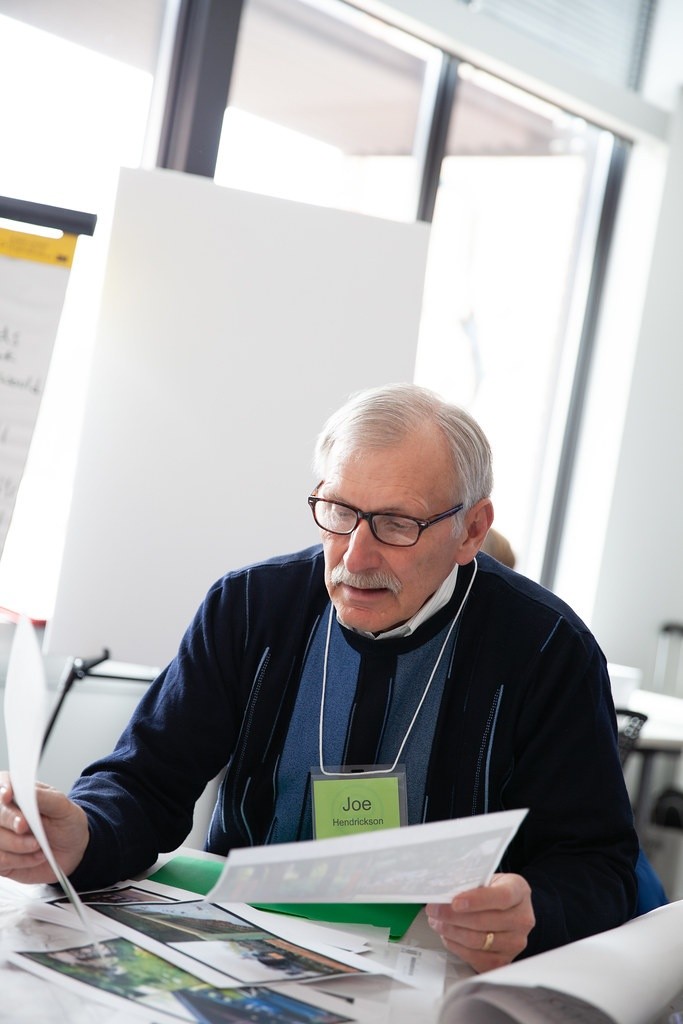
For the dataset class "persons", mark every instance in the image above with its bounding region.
[0,384,640,980]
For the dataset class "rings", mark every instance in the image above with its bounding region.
[481,932,494,952]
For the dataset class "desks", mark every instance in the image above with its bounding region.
[611,688,683,850]
[0,847,476,1024]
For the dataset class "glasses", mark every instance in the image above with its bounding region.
[308,479,464,547]
